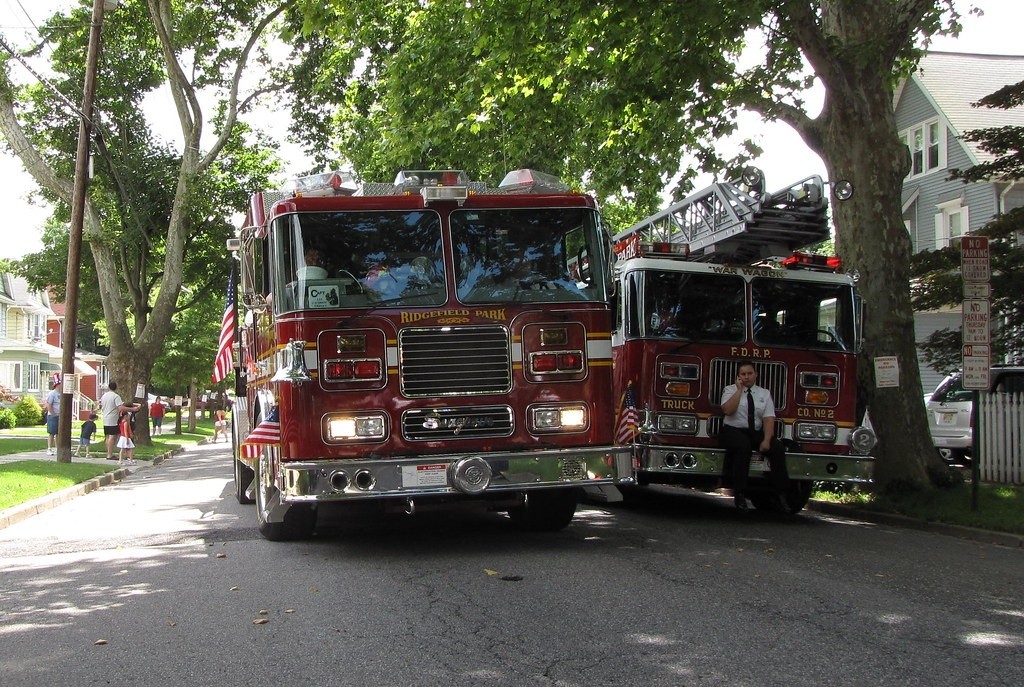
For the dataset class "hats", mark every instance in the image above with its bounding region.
[52,372,61,388]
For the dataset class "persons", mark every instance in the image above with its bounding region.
[212,405,228,443]
[150,396,165,436]
[74,414,96,458]
[265,245,329,308]
[720,361,792,512]
[116,412,137,464]
[101,382,140,459]
[46,380,62,455]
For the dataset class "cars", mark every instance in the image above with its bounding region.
[183,398,234,412]
[147,392,179,418]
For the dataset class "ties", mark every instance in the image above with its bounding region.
[746,388,755,433]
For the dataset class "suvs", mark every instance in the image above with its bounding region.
[923,364,1024,465]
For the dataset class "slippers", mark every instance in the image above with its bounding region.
[106,455,119,460]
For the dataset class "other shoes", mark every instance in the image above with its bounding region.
[128,460,137,465]
[778,496,791,512]
[47,449,54,456]
[212,440,216,443]
[75,452,81,457]
[117,461,126,465]
[86,454,93,458]
[734,487,747,509]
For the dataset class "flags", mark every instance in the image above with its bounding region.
[615,387,640,446]
[210,269,234,384]
[241,403,280,461]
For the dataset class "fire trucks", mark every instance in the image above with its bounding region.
[229,168,639,543]
[566,166,878,522]
